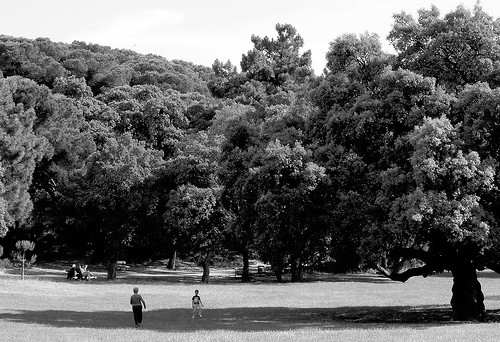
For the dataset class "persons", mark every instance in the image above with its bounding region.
[192,290,204,319]
[79,263,93,281]
[130,287,146,327]
[70,263,81,282]
